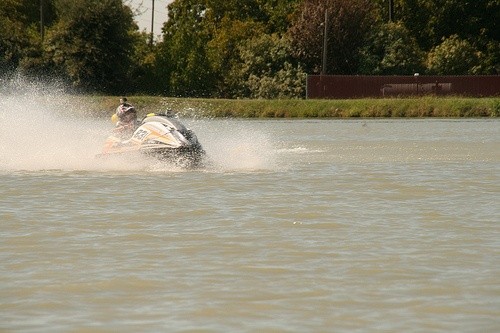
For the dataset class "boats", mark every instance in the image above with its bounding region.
[96,111,204,171]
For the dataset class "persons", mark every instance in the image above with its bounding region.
[115,97,137,127]
